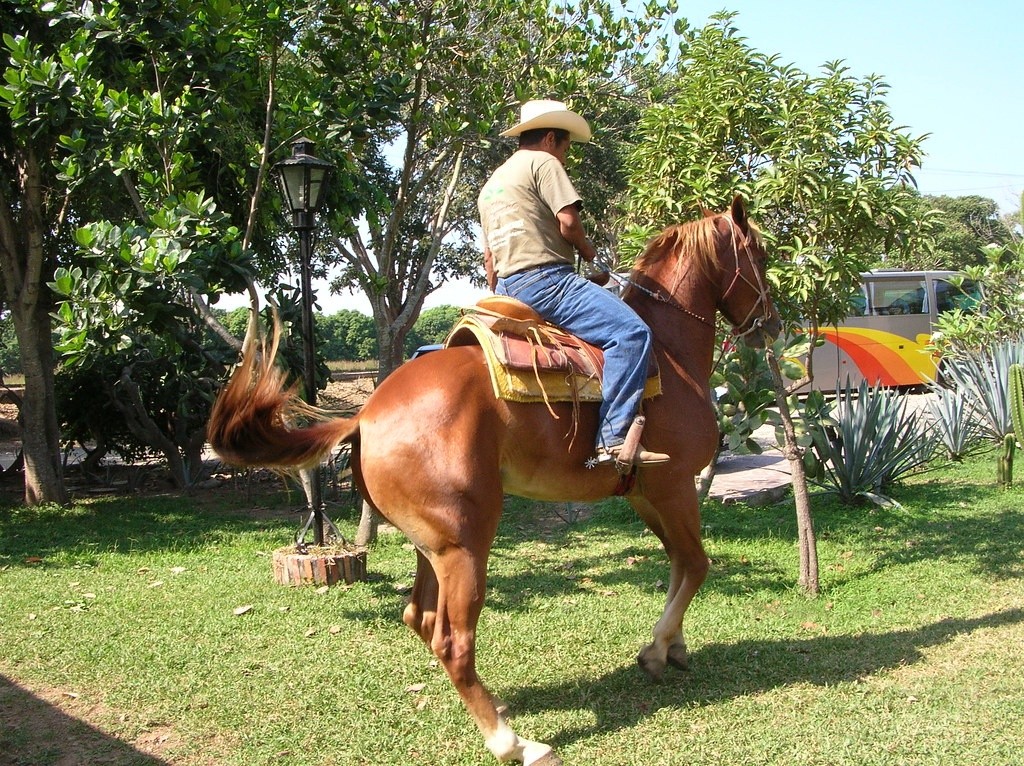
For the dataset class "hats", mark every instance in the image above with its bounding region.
[498,100,590,144]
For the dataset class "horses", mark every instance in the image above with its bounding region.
[205,194,781,766]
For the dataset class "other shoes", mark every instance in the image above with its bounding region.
[595,441,669,466]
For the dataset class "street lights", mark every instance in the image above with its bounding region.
[269,136,347,555]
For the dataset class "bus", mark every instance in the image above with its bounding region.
[714,268,986,398]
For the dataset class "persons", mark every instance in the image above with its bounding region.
[478,98,674,464]
